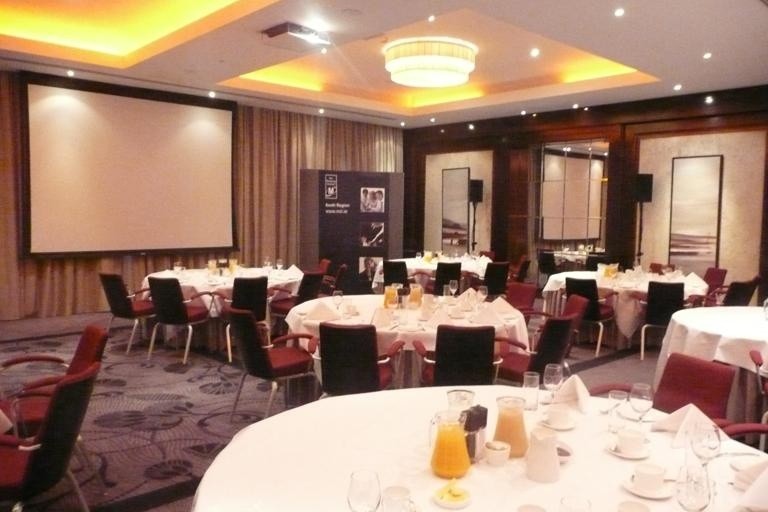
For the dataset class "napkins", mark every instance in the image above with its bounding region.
[457,288,486,302]
[485,296,517,312]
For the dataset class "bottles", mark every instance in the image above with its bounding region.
[471,404,488,460]
[458,408,480,463]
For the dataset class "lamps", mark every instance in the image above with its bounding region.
[630,174,653,266]
[377,36,478,91]
[468,179,483,251]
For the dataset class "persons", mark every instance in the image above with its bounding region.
[360,257,378,280]
[360,189,384,212]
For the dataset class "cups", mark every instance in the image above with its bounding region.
[168,250,288,286]
[607,390,627,432]
[617,430,646,454]
[522,370,540,410]
[593,262,685,286]
[675,460,712,511]
[324,277,510,327]
[410,248,482,265]
[447,389,475,422]
[631,463,667,490]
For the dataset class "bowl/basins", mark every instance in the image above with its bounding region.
[433,483,476,509]
[557,442,573,464]
[484,441,512,468]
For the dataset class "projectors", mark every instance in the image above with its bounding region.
[261,22,331,53]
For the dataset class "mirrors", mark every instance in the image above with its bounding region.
[537,140,608,272]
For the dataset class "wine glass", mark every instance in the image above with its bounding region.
[544,364,564,403]
[691,421,721,495]
[629,381,654,430]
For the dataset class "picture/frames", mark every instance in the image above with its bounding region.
[666,154,722,278]
[442,167,470,256]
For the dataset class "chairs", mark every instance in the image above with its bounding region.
[99,258,347,366]
[370,253,531,298]
[226,309,318,420]
[586,350,733,430]
[1,324,113,497]
[724,410,768,454]
[319,321,404,399]
[498,315,576,393]
[0,362,101,511]
[411,323,505,387]
[542,262,761,360]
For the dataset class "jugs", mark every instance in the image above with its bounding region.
[493,395,528,459]
[429,407,470,480]
[525,426,559,482]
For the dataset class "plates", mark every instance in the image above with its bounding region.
[604,439,656,461]
[616,405,660,422]
[623,474,677,500]
[539,415,575,432]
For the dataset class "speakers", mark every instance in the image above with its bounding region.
[468,180,483,202]
[633,174,653,202]
